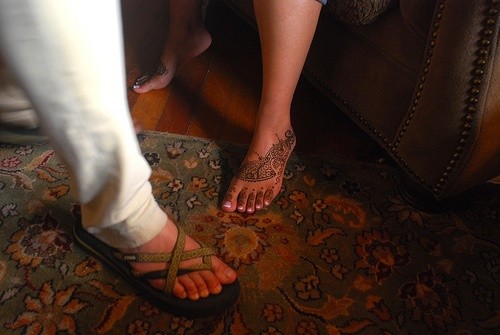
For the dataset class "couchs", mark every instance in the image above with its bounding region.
[225,0,500,213]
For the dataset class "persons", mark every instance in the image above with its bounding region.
[1,0,244,324]
[130,0,327,215]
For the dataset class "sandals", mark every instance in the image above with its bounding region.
[73,207,242,314]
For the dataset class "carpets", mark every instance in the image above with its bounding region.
[0,129,500,335]
